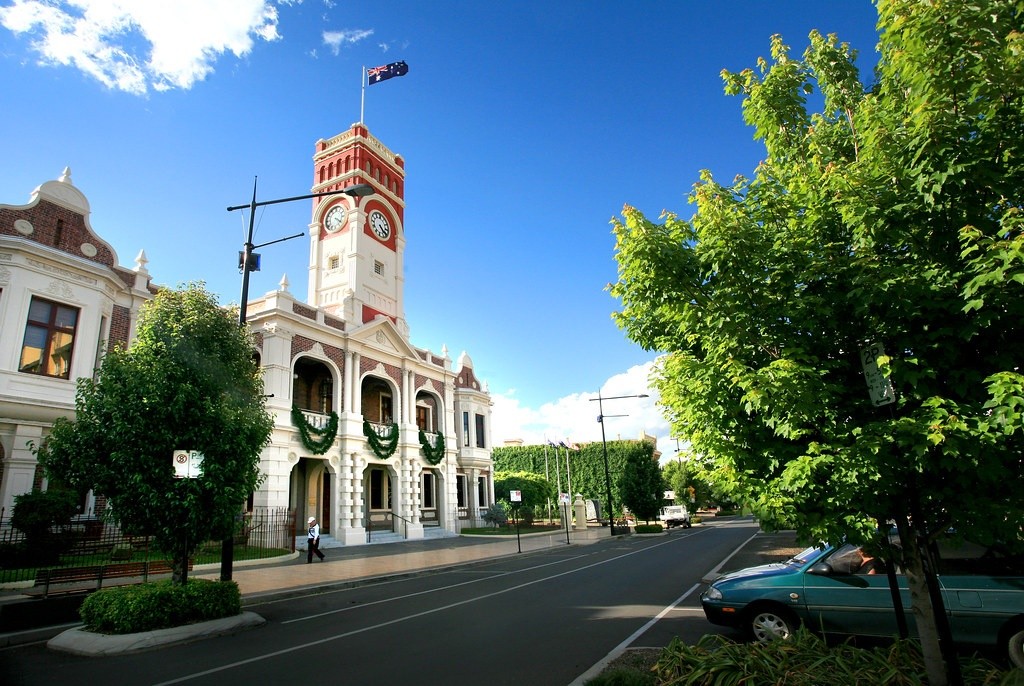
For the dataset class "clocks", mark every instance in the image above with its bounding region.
[323,204,349,233]
[369,209,392,242]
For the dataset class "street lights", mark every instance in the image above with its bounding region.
[670,437,681,466]
[588,385,650,536]
[219,175,376,581]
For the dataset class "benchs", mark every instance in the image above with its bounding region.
[34,557,193,594]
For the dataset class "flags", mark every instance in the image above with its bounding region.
[366,60,408,85]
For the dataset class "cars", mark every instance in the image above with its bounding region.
[699,523,1024,672]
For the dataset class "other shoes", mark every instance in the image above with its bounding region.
[321,555,325,561]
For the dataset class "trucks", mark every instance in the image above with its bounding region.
[658,504,692,529]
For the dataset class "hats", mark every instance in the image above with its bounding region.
[307,517,315,524]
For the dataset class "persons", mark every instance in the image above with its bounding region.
[303,517,327,564]
[856,542,885,575]
